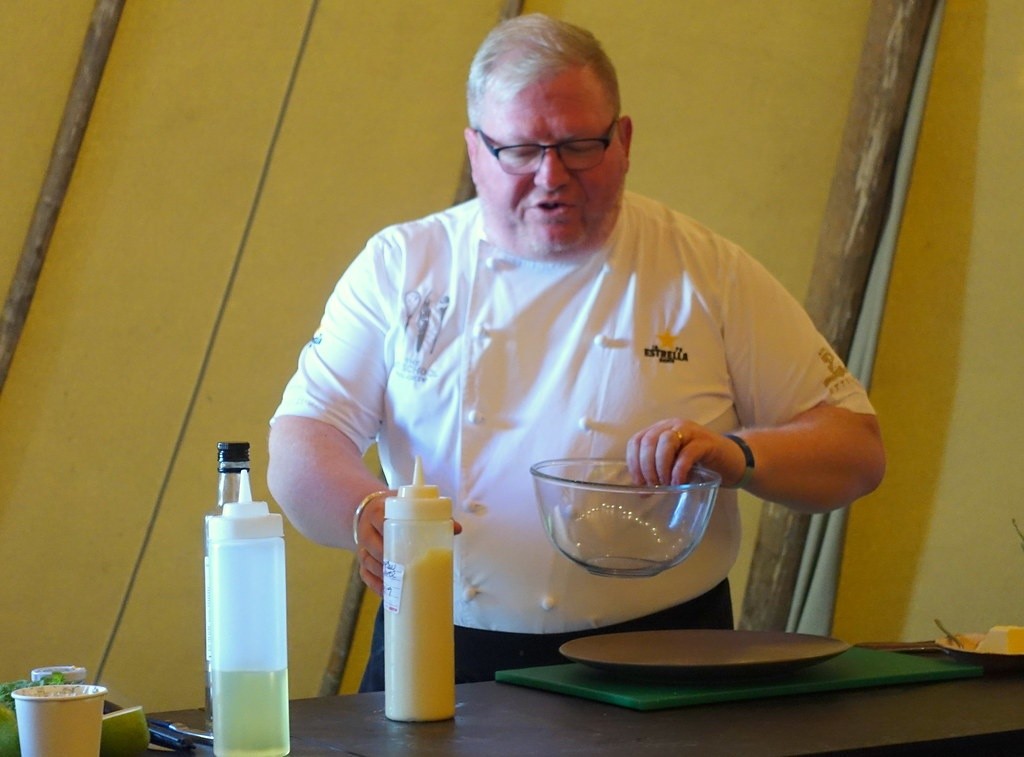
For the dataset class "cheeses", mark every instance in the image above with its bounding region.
[973,625,1024,655]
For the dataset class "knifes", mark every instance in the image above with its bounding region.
[150,719,214,743]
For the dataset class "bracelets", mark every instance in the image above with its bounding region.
[722,433,755,489]
[353,491,385,545]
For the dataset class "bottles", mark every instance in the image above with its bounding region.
[202,440,253,732]
[383,456,455,722]
[210,470,290,757]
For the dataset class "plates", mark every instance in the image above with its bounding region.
[558,629,855,678]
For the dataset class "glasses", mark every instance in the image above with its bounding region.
[474,119,618,175]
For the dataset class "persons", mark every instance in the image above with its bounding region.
[267,12,885,692]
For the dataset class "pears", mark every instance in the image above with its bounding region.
[0,704,22,757]
[100,706,151,757]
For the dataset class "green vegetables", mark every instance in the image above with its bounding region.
[0,671,63,710]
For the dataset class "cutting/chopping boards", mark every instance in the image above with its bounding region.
[494,648,985,711]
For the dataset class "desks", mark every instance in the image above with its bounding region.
[145,639,1024,757]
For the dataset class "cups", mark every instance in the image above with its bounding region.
[11,684,109,756]
[100,706,150,757]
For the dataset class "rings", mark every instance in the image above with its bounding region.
[671,427,683,445]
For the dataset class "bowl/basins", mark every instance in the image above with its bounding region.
[935,634,1024,672]
[530,457,722,580]
